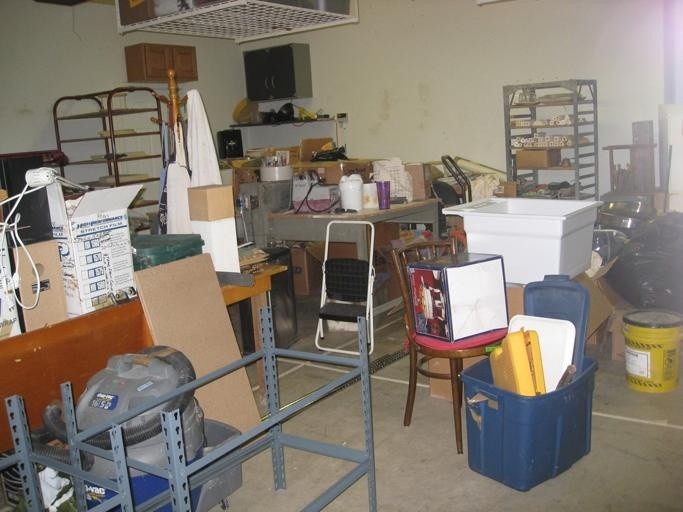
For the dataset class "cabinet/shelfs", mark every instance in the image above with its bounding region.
[242,41,313,106]
[502,81,598,199]
[52,88,166,209]
[126,41,204,84]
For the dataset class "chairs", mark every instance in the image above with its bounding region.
[312,218,381,355]
[390,230,473,456]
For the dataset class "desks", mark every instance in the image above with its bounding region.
[270,200,443,264]
[220,265,288,406]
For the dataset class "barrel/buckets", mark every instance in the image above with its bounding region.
[622,309,683,393]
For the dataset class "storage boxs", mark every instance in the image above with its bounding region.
[188,183,236,221]
[457,273,609,492]
[411,250,512,343]
[443,196,603,286]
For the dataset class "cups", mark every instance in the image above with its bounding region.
[362,182,379,211]
[375,181,391,210]
